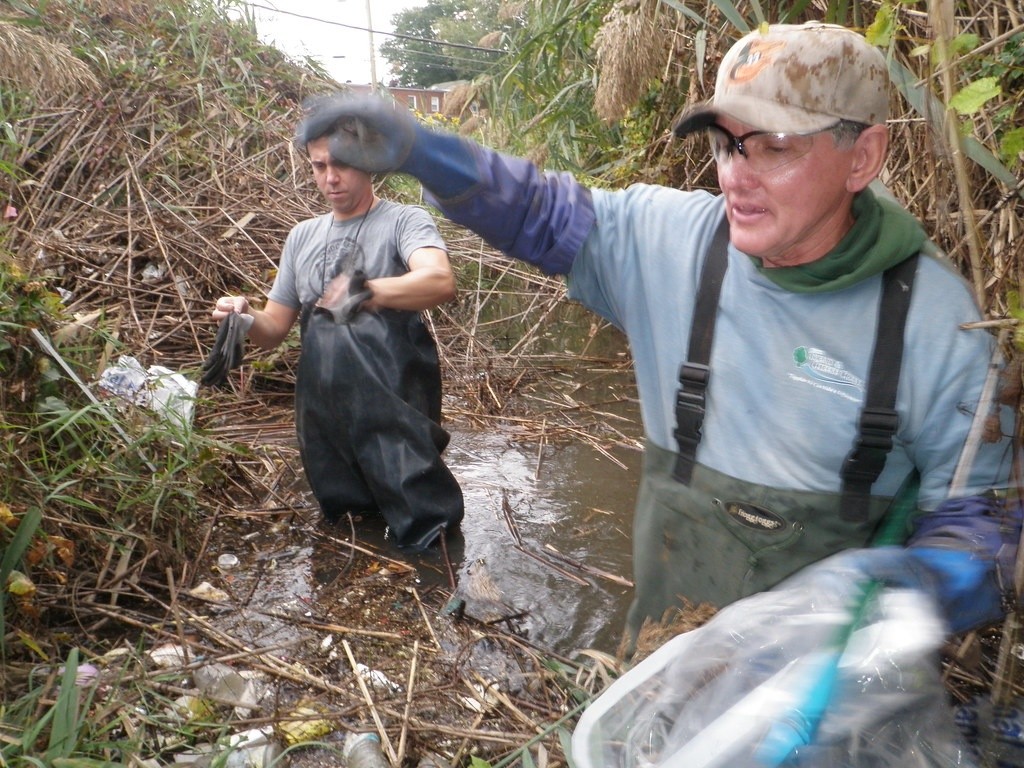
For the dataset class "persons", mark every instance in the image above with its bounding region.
[293,19,1024,768]
[212,126,463,543]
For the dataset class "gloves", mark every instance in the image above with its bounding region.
[314,270,374,324]
[868,496,1022,634]
[299,93,594,275]
[202,308,255,387]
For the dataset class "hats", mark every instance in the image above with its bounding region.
[674,20,890,135]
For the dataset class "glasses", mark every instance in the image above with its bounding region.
[706,122,870,173]
